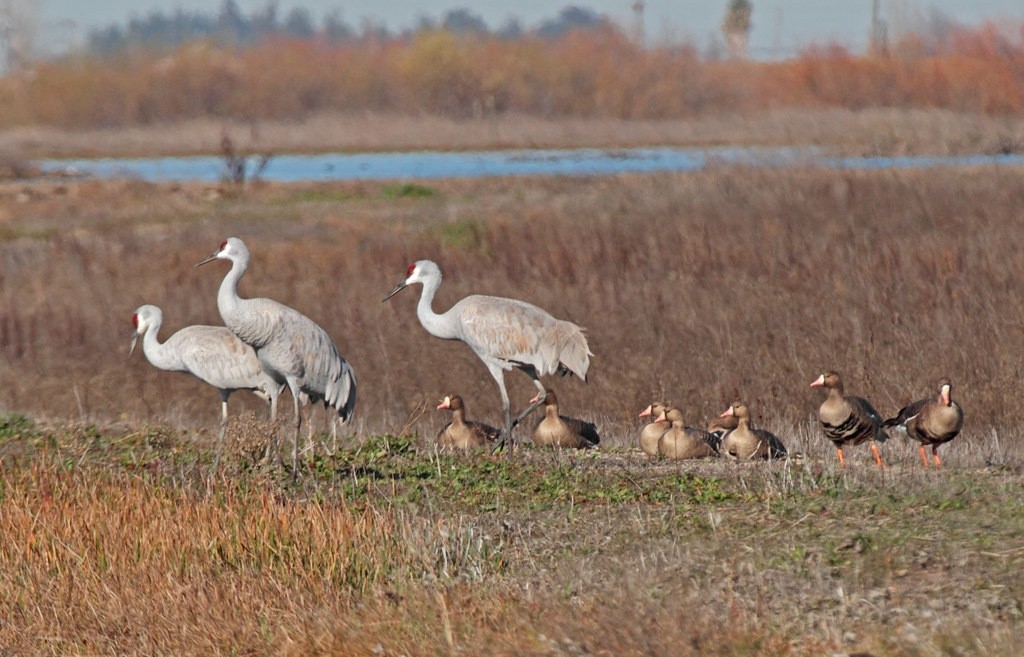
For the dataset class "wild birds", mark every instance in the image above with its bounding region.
[808,371,963,470]
[130,236,356,490]
[380,257,599,452]
[636,400,787,460]
